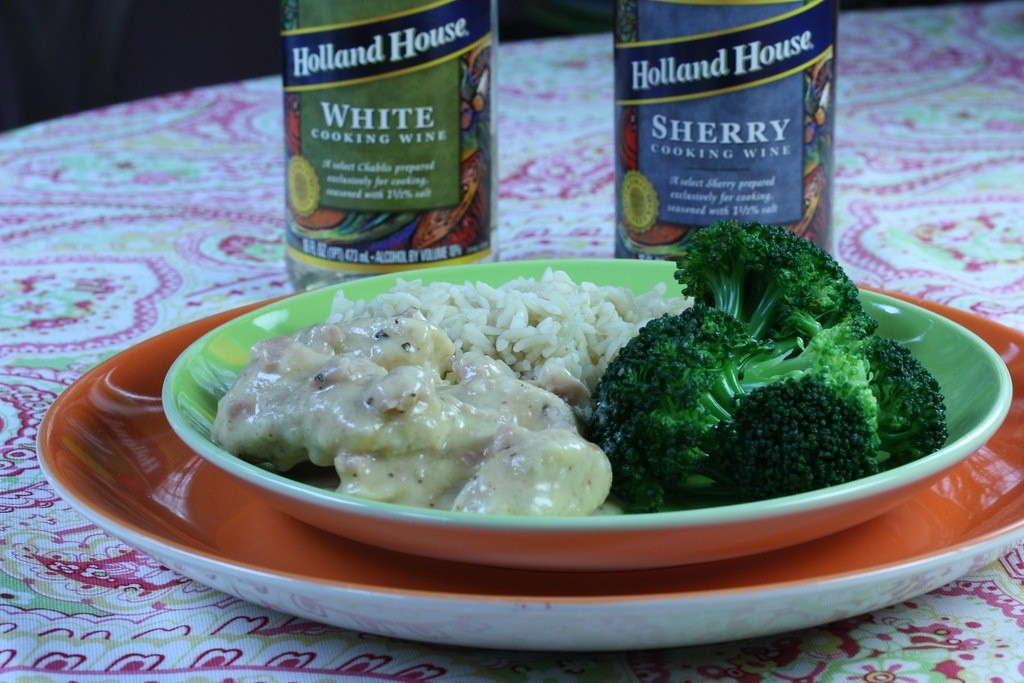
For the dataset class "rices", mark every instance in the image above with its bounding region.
[325,268,694,394]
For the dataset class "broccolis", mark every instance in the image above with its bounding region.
[575,217,949,520]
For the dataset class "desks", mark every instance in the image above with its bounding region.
[0,0,1024,683]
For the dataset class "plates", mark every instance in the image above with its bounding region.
[36,284,1024,649]
[162,259,1014,572]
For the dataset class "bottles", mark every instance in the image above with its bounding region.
[613,0,835,257]
[279,0,495,291]
